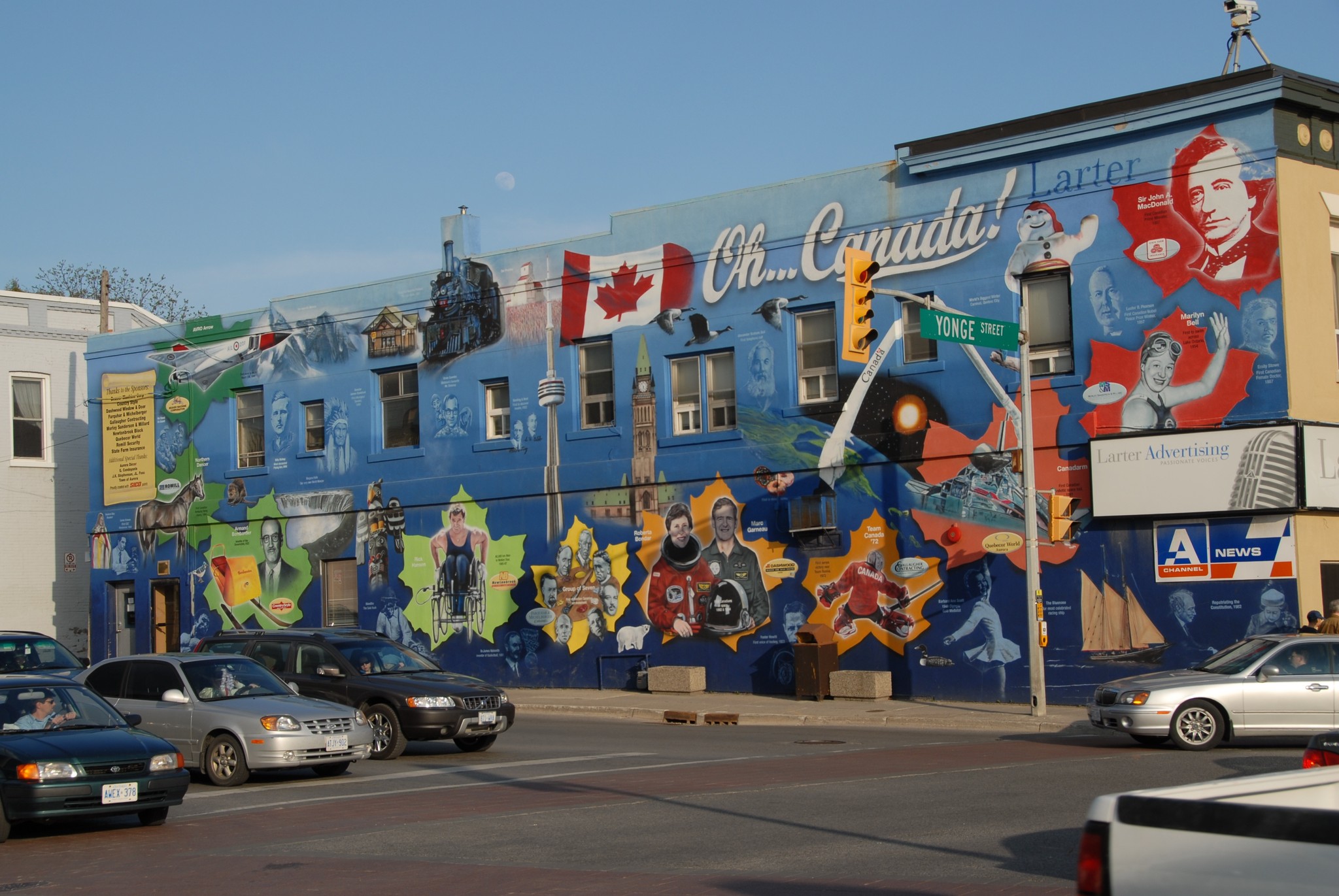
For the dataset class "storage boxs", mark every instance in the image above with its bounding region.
[794,623,835,644]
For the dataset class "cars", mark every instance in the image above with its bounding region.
[1085,633,1339,752]
[1302,730,1339,768]
[71,652,373,787]
[0,674,190,843]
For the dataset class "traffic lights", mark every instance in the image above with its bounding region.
[841,247,881,364]
[1047,495,1081,543]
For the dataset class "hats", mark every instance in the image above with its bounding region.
[1307,610,1326,620]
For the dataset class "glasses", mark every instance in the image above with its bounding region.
[45,698,55,705]
[360,660,371,667]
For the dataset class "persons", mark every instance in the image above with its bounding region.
[111,536,212,653]
[356,653,404,675]
[738,340,783,411]
[14,688,75,730]
[376,497,1021,702]
[269,392,356,478]
[256,518,300,597]
[1087,124,1282,436]
[1244,587,1298,639]
[1289,600,1339,674]
[198,667,261,699]
[1160,588,1218,668]
[429,393,540,452]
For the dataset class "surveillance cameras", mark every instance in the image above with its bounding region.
[1223,0,1258,29]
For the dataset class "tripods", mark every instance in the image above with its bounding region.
[1221,27,1270,75]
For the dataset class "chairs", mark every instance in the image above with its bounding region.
[253,642,286,672]
[4,689,34,724]
[350,649,363,673]
[4,646,37,671]
[230,642,246,654]
[1311,643,1328,670]
[301,648,320,674]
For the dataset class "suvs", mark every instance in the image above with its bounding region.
[0,629,91,681]
[192,627,516,760]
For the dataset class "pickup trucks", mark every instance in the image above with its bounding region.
[1076,762,1339,896]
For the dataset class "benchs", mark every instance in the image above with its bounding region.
[647,665,707,696]
[829,670,893,703]
